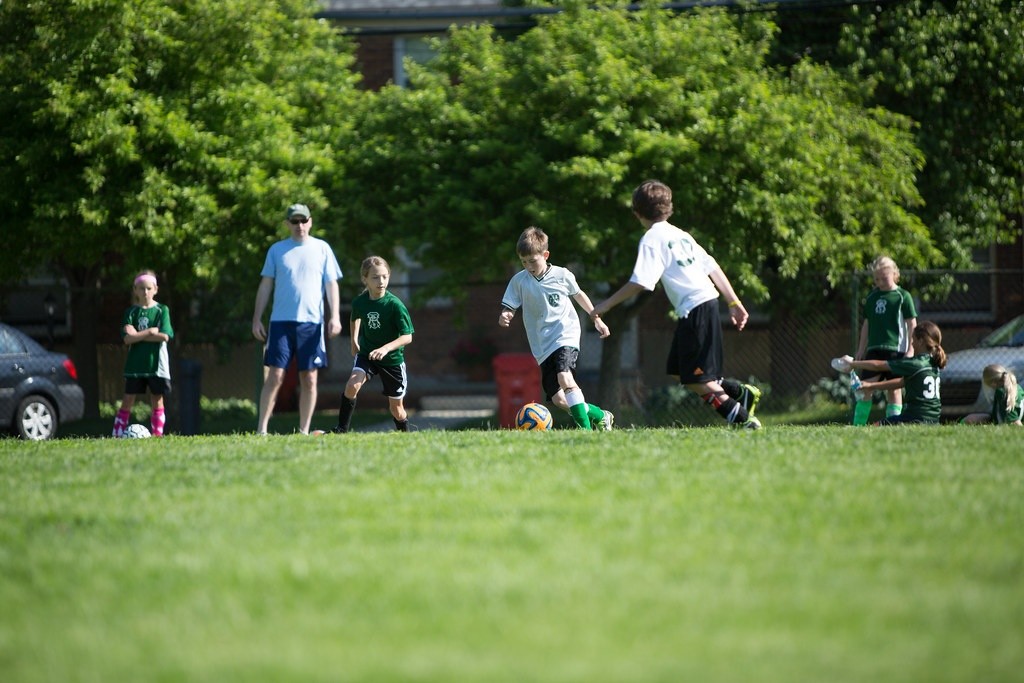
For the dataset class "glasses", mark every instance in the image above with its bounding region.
[289,219,308,224]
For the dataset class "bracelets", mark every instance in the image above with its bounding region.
[728,300,741,309]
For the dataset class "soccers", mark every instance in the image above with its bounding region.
[514,402,553,433]
[121,423,151,439]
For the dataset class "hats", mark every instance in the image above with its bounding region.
[288,204,310,220]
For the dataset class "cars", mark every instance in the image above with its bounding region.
[0,322,85,440]
[940,313,1024,424]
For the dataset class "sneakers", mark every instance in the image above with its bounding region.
[743,417,761,429]
[735,384,761,416]
[593,410,614,432]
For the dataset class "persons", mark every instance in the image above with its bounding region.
[498,226,614,432]
[841,322,947,426]
[959,364,1024,426]
[251,204,344,437]
[111,269,174,439]
[323,255,415,436]
[853,256,918,427]
[589,179,763,432]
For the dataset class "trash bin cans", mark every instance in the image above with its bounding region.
[162,361,202,434]
[493,353,541,429]
